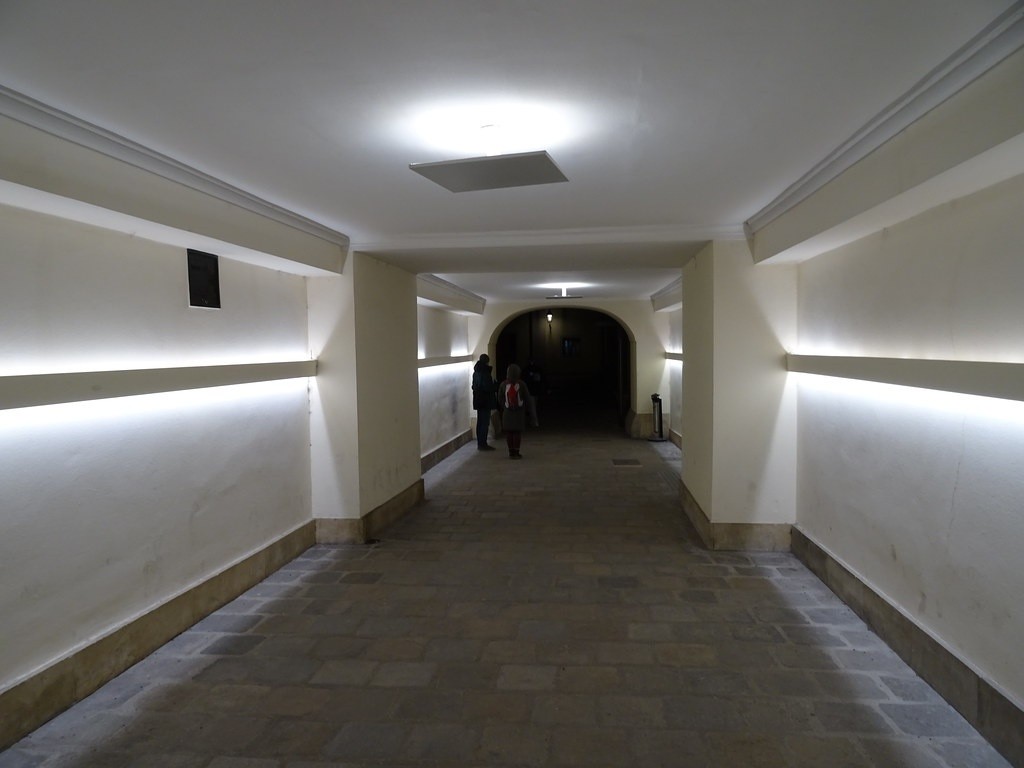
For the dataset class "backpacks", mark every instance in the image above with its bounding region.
[502,379,525,409]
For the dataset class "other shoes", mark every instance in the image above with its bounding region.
[509,449,514,456]
[513,450,521,458]
[478,445,494,451]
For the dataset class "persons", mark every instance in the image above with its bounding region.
[497,364,529,460]
[472,354,498,451]
[524,357,541,429]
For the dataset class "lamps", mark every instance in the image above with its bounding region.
[545,310,553,333]
